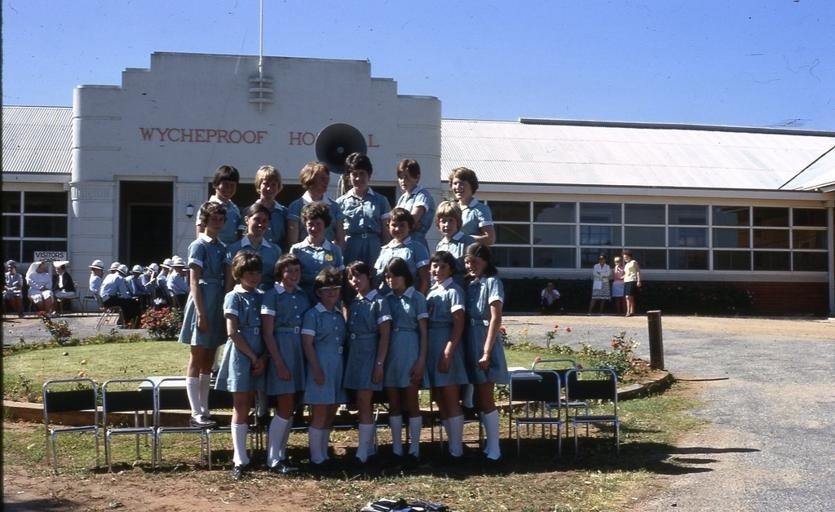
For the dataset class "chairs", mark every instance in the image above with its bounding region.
[509,369,563,461]
[57,282,82,317]
[431,389,483,457]
[89,288,127,332]
[83,287,100,317]
[262,408,434,463]
[42,378,99,473]
[532,360,588,440]
[155,279,182,310]
[206,379,257,470]
[102,379,156,474]
[566,369,621,458]
[155,378,206,471]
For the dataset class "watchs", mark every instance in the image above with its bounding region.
[374,358,386,368]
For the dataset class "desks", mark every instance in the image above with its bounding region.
[135,373,230,460]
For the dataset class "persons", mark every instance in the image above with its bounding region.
[286,162,346,250]
[289,202,345,282]
[461,241,511,471]
[258,253,311,476]
[448,166,496,247]
[240,164,289,245]
[373,207,431,294]
[338,260,392,477]
[621,248,641,317]
[213,250,270,483]
[99,255,190,330]
[2,259,26,319]
[178,200,228,428]
[195,164,242,246]
[435,200,475,288]
[51,261,76,319]
[88,260,104,296]
[391,158,436,258]
[585,253,611,316]
[335,151,392,268]
[223,202,281,295]
[540,280,565,315]
[300,267,349,477]
[610,255,625,315]
[25,260,58,319]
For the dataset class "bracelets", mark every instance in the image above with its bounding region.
[636,279,642,283]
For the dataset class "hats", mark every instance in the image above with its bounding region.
[146,263,160,275]
[159,259,172,269]
[110,262,121,271]
[184,262,189,270]
[172,257,179,262]
[89,260,105,271]
[117,265,129,277]
[130,266,144,276]
[171,259,184,267]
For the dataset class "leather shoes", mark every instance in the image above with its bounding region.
[188,414,218,428]
[386,452,423,471]
[474,443,506,472]
[269,461,300,475]
[231,465,243,482]
[309,459,351,474]
[241,465,260,477]
[349,451,382,471]
[430,441,470,469]
